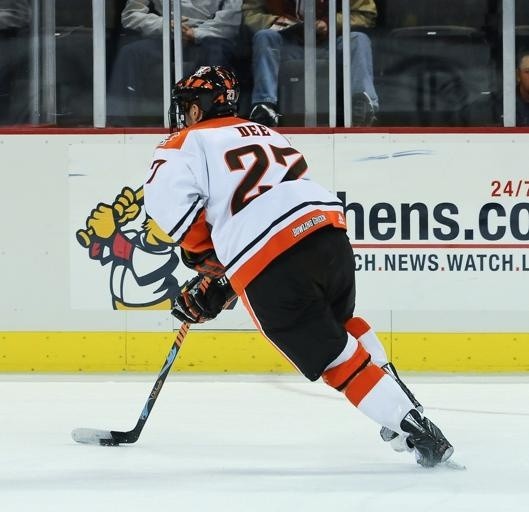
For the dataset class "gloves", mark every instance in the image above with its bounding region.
[171,250,238,324]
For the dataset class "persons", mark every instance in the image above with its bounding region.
[142,65,454,470]
[0,0,529,128]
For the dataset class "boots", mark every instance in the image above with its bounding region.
[370,362,424,444]
[398,415,454,469]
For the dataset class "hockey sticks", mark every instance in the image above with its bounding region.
[71,277,211,445]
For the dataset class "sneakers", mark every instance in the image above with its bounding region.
[247,103,280,128]
[346,89,378,130]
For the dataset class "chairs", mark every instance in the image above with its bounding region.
[0,0,529,122]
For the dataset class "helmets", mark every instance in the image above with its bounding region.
[173,64,244,117]
